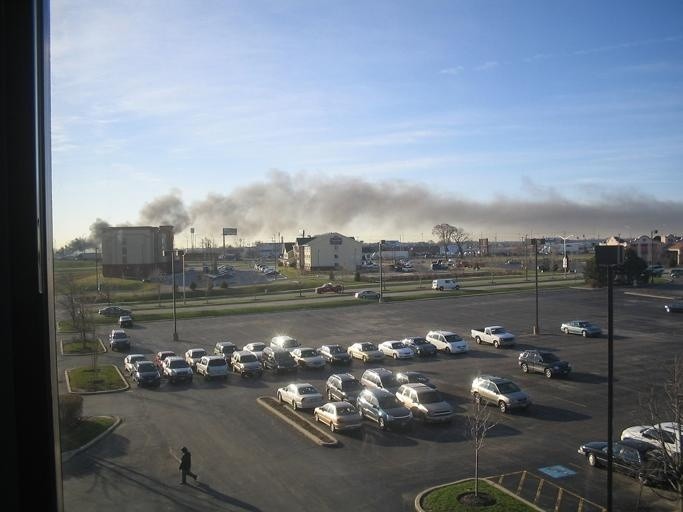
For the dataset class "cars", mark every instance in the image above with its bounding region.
[217,263,234,282]
[581,437,676,487]
[664,297,682,312]
[506,258,520,264]
[118,315,134,328]
[561,319,600,338]
[431,259,478,271]
[393,262,415,273]
[98,307,132,316]
[354,289,380,299]
[252,263,280,278]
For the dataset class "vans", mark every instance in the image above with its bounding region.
[431,279,460,292]
[648,264,664,273]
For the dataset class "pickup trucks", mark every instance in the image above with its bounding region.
[314,283,344,295]
[471,325,515,349]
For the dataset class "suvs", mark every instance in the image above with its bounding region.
[622,421,683,454]
[470,374,529,413]
[519,351,573,379]
[669,269,682,279]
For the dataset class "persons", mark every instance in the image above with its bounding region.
[178,446,197,485]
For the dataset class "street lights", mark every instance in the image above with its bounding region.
[379,239,386,302]
[651,230,659,285]
[94,247,99,293]
[162,248,185,342]
[595,242,625,512]
[556,234,574,280]
[530,238,547,335]
